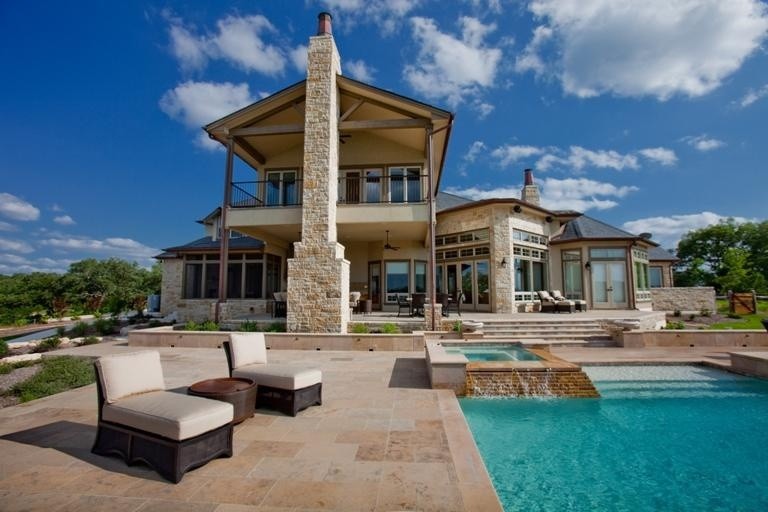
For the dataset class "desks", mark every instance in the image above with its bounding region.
[188,376,258,424]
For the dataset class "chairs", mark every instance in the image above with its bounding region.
[266,290,372,319]
[549,290,588,312]
[396,288,464,317]
[536,290,576,314]
[91,348,236,483]
[224,331,324,416]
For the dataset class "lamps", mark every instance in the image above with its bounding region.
[501,256,508,268]
[584,261,591,271]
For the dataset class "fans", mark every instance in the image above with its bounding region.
[339,134,352,145]
[377,231,401,252]
